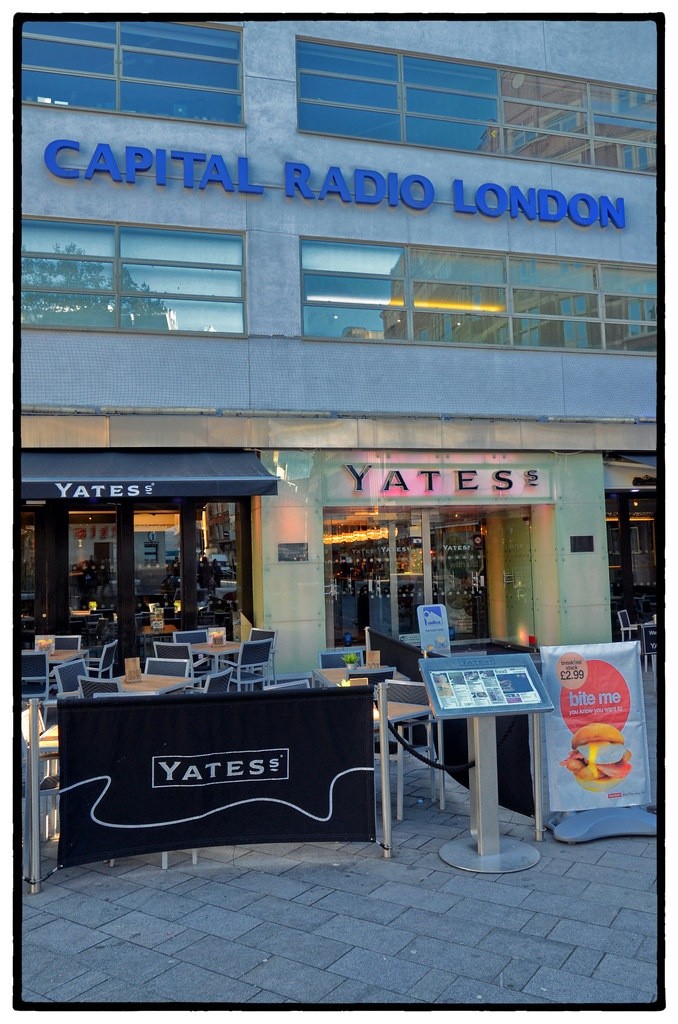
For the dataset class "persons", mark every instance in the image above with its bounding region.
[76,555,114,610]
[172,557,222,589]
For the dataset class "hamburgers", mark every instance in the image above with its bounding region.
[560,722,632,792]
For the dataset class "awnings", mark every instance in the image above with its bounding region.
[20,451,281,501]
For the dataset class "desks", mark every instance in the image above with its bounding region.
[114,673,202,696]
[26,725,61,841]
[641,620,656,673]
[191,641,241,668]
[311,666,410,688]
[373,702,437,803]
[22,650,90,682]
[135,624,178,662]
[175,605,208,618]
[71,610,91,617]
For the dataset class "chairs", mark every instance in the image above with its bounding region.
[20,588,238,646]
[22,628,438,821]
[617,609,643,642]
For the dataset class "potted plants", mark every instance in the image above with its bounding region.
[340,652,361,670]
